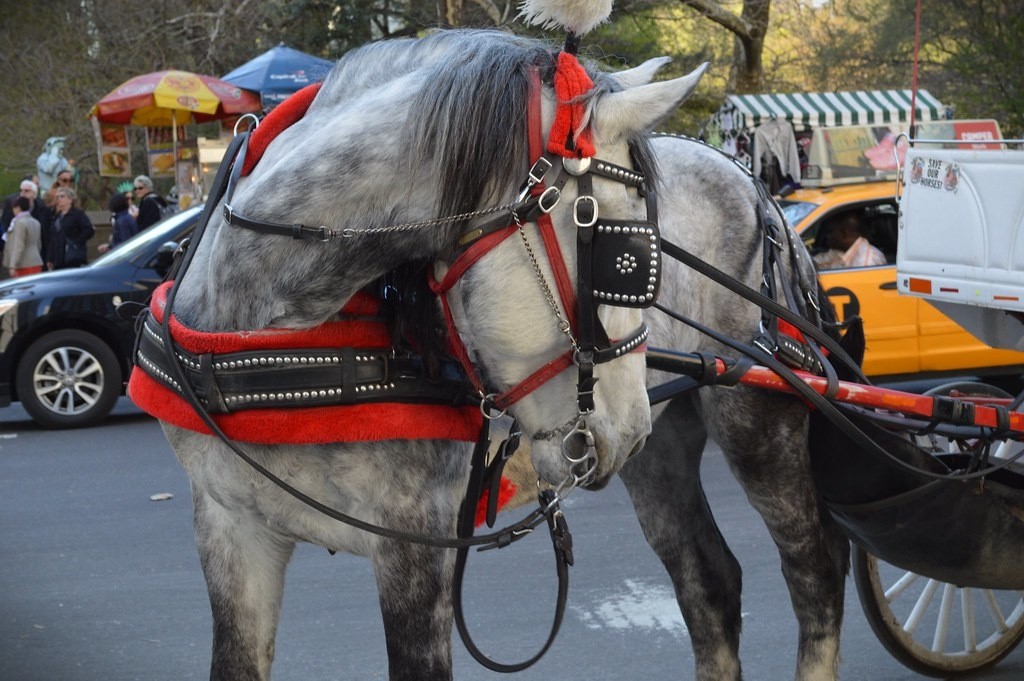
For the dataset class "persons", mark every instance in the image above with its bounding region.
[812,215,888,271]
[36,136,68,199]
[0,174,180,280]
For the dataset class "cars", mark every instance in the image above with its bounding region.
[764,174,1023,382]
[0,201,206,431]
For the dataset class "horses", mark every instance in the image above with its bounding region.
[124,25,854,681]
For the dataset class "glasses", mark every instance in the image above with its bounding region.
[56,194,65,200]
[20,189,29,192]
[126,195,136,201]
[58,177,74,182]
[134,186,145,191]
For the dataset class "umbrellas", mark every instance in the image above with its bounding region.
[83,42,333,182]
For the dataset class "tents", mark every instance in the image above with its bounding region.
[717,88,946,136]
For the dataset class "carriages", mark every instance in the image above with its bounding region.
[126,0,1024,680]
